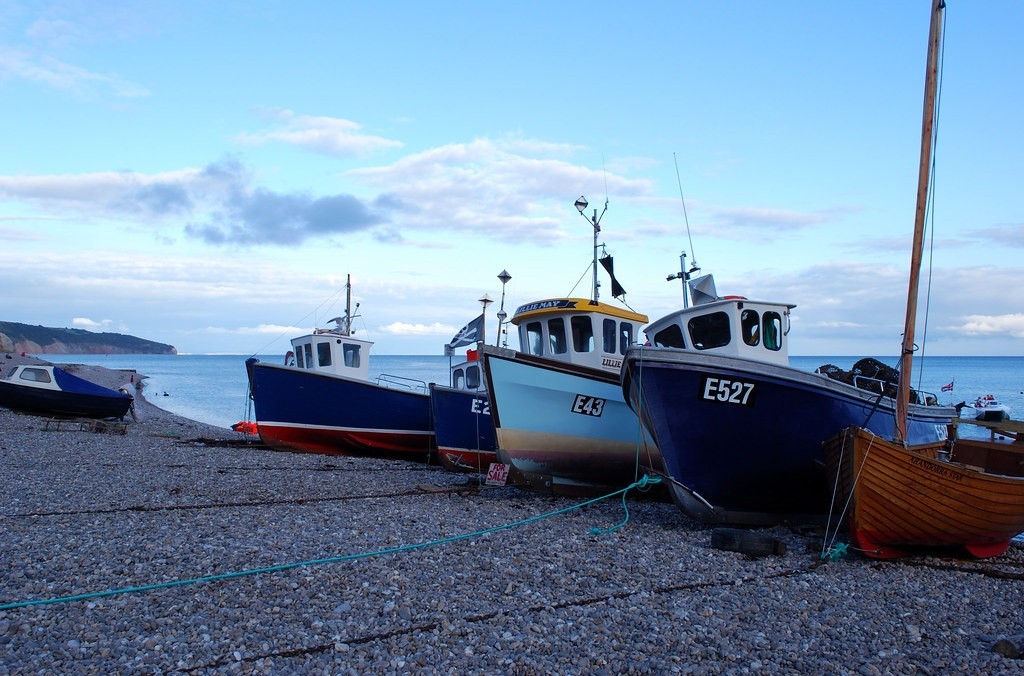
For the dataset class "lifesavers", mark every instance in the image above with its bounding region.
[285,351,295,367]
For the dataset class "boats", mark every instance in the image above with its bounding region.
[968,394,1012,423]
[477,195,676,503]
[619,148,966,530]
[243,272,441,464]
[429,266,512,481]
[0,363,135,421]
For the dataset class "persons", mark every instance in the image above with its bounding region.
[975,395,995,407]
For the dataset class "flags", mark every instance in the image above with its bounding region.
[941,381,953,392]
[451,314,485,348]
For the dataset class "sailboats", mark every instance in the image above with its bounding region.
[820,0,1024,563]
